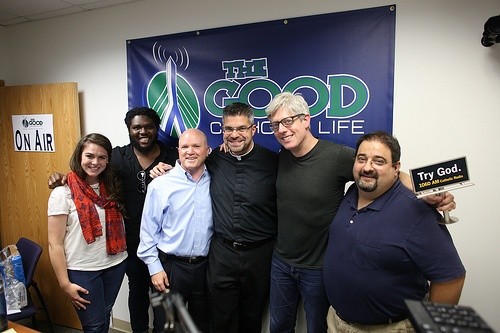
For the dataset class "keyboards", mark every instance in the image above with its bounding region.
[403,298,494,333]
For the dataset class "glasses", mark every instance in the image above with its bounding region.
[269,113,305,132]
[137,170,146,194]
[222,124,253,132]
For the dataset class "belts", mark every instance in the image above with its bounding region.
[174,257,206,265]
[216,235,271,251]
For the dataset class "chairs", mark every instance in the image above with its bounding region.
[7,238,57,333]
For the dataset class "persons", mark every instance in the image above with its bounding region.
[138,128,213,333]
[48,106,177,333]
[324,131,466,333]
[220,92,456,333]
[150,102,278,333]
[33,133,128,333]
